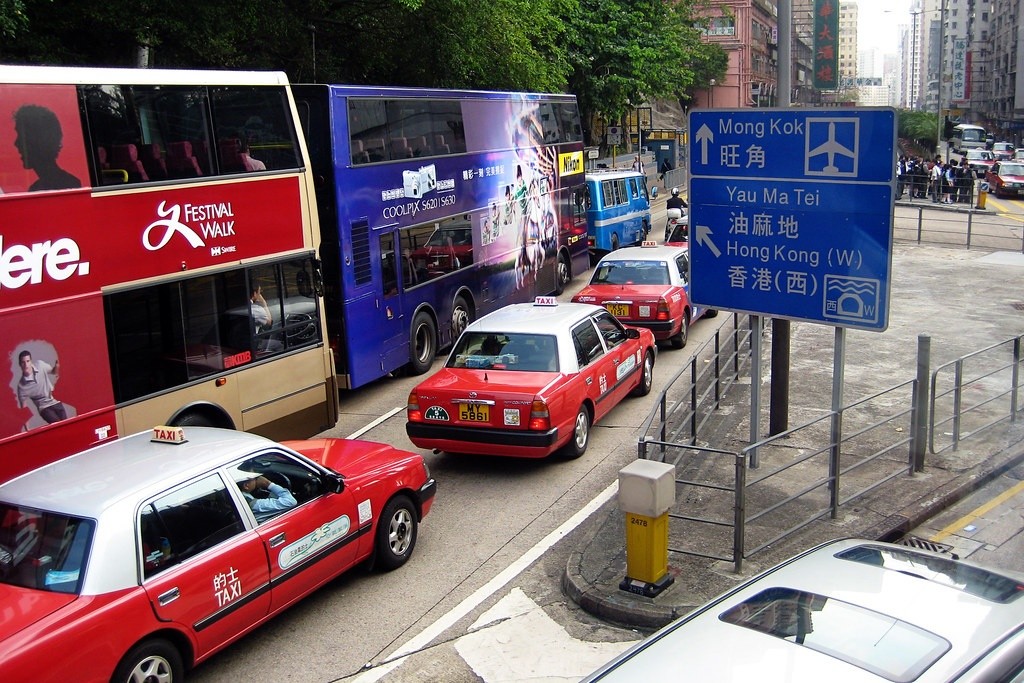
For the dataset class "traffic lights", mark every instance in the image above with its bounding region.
[943,115,959,139]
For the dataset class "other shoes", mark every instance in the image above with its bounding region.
[895,192,957,204]
[658,179,662,182]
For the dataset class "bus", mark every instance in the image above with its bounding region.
[290,80,591,393]
[0,60,344,529]
[584,168,659,267]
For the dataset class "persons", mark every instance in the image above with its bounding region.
[895,153,977,204]
[483,165,529,242]
[242,147,266,171]
[661,158,671,188]
[632,156,644,173]
[403,240,439,279]
[18,351,67,423]
[665,188,687,240]
[237,462,297,523]
[249,279,282,352]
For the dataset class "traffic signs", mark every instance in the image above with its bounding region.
[682,106,897,333]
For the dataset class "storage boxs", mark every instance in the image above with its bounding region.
[667,208,682,219]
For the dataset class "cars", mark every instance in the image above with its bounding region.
[0,424,440,683]
[949,123,1024,176]
[404,295,657,461]
[665,215,689,249]
[984,161,1024,199]
[568,239,717,351]
[575,533,1024,683]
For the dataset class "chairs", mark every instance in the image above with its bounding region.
[98,138,256,185]
[351,134,451,164]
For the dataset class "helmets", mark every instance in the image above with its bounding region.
[671,187,679,197]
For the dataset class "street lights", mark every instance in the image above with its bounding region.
[804,50,826,104]
[709,79,717,108]
[306,23,317,83]
[883,7,950,112]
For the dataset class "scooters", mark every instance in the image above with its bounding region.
[664,196,688,241]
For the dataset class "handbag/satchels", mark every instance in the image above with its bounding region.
[937,173,942,180]
